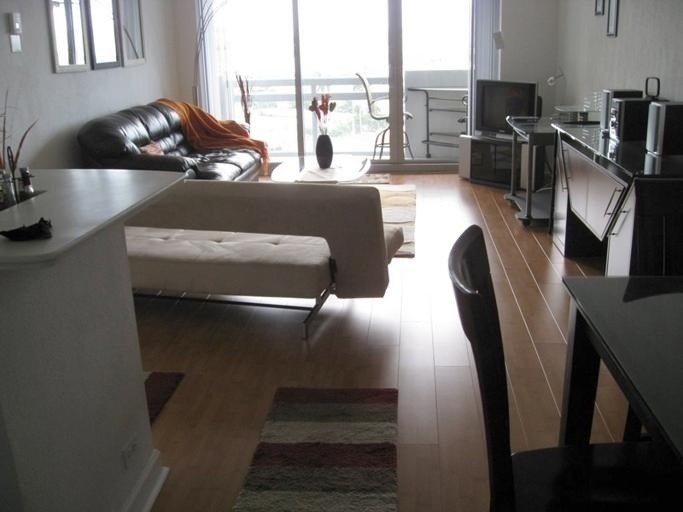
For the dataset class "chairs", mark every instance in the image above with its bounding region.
[356,73,416,159]
[450,225,683,511]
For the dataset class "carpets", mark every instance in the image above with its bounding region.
[143,370,183,422]
[232,384,401,511]
[377,185,417,258]
[337,172,391,184]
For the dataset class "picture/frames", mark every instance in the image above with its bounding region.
[594,0,604,15]
[116,1,147,67]
[84,0,121,69]
[46,0,91,72]
[606,0,617,38]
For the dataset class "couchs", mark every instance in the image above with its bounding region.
[79,98,265,182]
[125,179,405,324]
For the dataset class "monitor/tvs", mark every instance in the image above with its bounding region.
[474,79,542,142]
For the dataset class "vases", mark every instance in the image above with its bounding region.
[317,135,333,169]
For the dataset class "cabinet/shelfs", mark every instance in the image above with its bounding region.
[552,123,683,274]
[459,134,545,192]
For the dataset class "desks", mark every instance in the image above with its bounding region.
[0,169,189,510]
[409,86,469,158]
[562,275,682,466]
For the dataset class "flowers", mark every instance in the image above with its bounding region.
[309,94,336,134]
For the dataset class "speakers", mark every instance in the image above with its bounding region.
[599,90,642,131]
[645,101,683,158]
